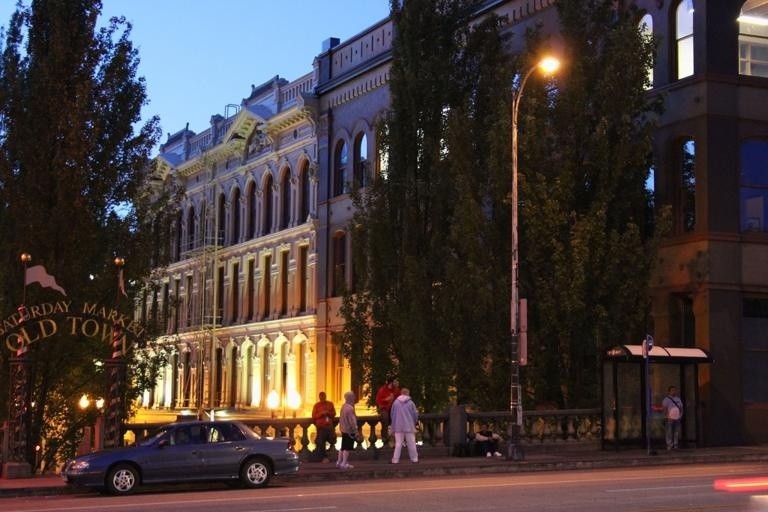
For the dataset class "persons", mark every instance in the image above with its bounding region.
[389,388,419,464]
[376,376,401,442]
[475,424,502,458]
[336,391,359,470]
[312,392,337,463]
[662,385,685,454]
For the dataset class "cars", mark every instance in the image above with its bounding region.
[174,408,226,421]
[60,418,303,493]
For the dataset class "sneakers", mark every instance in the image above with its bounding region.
[494,451,507,457]
[335,463,355,470]
[486,451,492,458]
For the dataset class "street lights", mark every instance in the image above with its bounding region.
[81,388,105,448]
[285,390,302,418]
[267,390,280,418]
[506,54,574,461]
